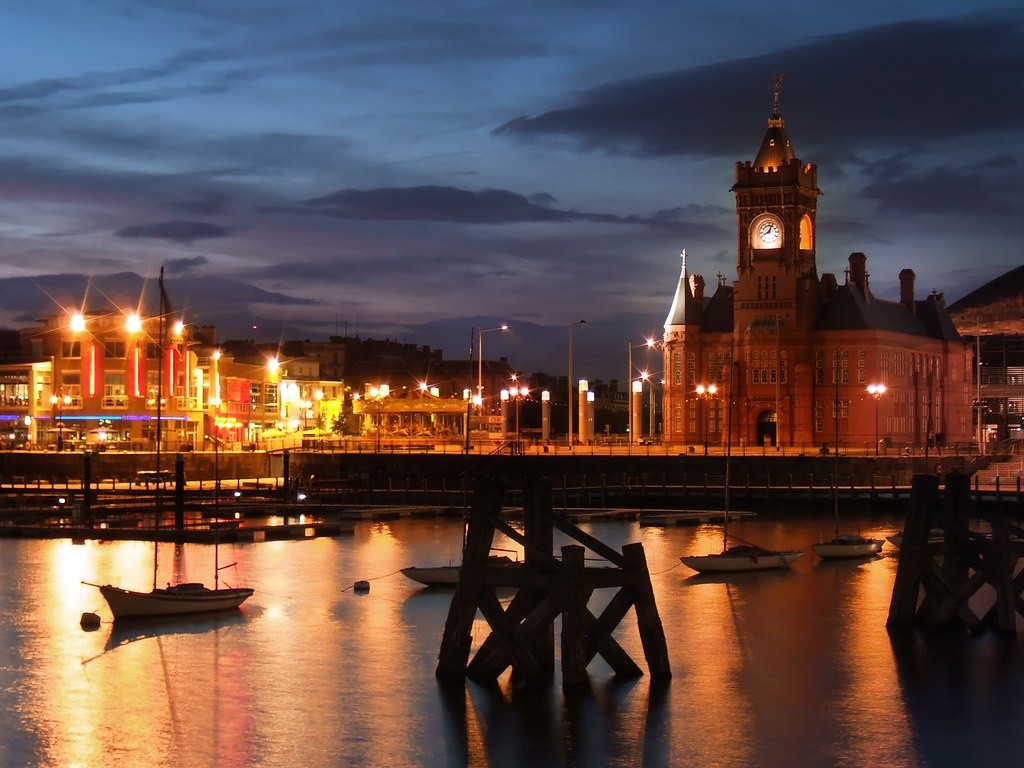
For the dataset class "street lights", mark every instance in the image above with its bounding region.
[370,387,389,452]
[868,384,885,456]
[50,387,72,453]
[478,324,508,419]
[694,384,718,455]
[628,339,654,444]
[567,318,586,449]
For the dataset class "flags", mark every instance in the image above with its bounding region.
[161,283,174,328]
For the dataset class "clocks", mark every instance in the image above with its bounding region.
[759,223,779,242]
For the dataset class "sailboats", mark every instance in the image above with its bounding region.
[398,326,523,586]
[813,348,885,560]
[81,267,253,619]
[677,336,806,574]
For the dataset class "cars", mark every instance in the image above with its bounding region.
[973,410,1021,444]
